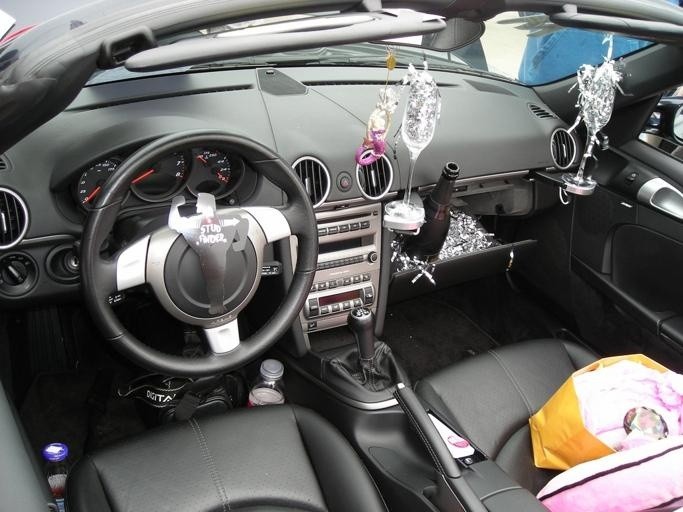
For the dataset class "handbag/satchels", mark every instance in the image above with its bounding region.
[128,374,248,429]
[527,353,682,470]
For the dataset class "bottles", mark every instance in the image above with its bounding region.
[407,161,461,265]
[245,360,288,408]
[42,440,74,511]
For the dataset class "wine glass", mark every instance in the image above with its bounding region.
[563,78,618,187]
[386,86,438,218]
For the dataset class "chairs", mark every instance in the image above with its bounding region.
[64,400,382,509]
[412,336,605,512]
